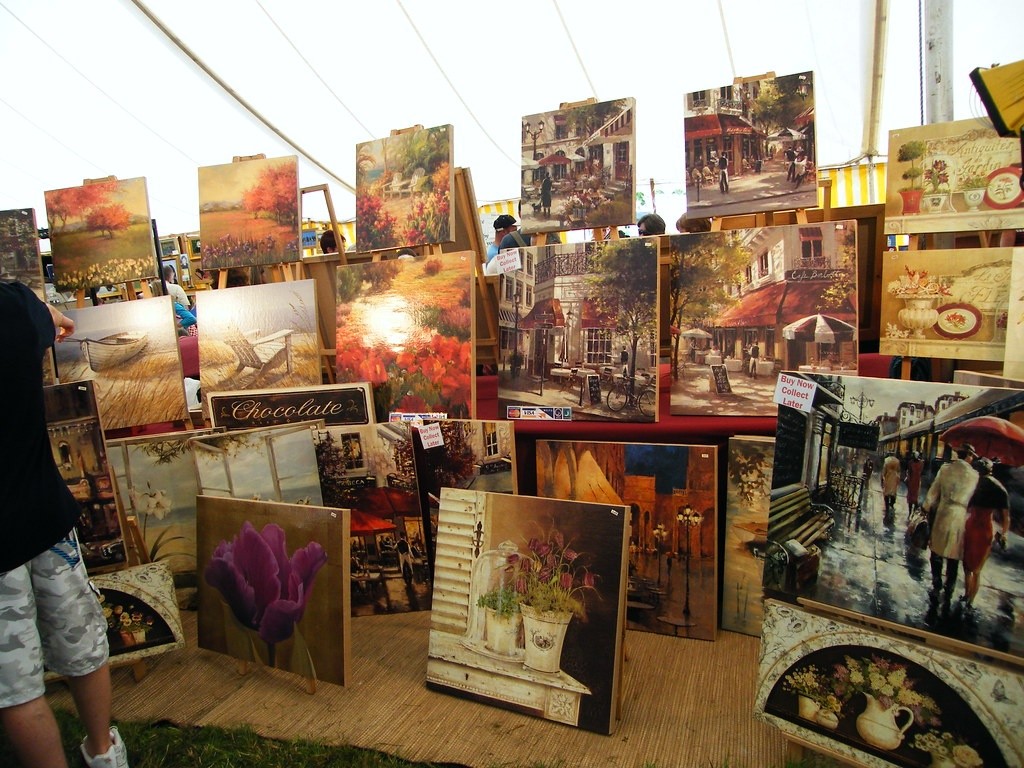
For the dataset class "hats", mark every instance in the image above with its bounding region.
[493,215,521,231]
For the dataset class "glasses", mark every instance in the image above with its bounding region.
[638,229,649,236]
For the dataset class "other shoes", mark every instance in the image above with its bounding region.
[79,726,127,768]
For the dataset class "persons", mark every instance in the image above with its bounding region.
[148,280,197,328]
[675,211,712,233]
[209,267,250,289]
[0,281,129,768]
[68,264,191,310]
[485,213,522,267]
[320,230,347,254]
[497,199,562,254]
[637,213,666,238]
[178,327,202,404]
